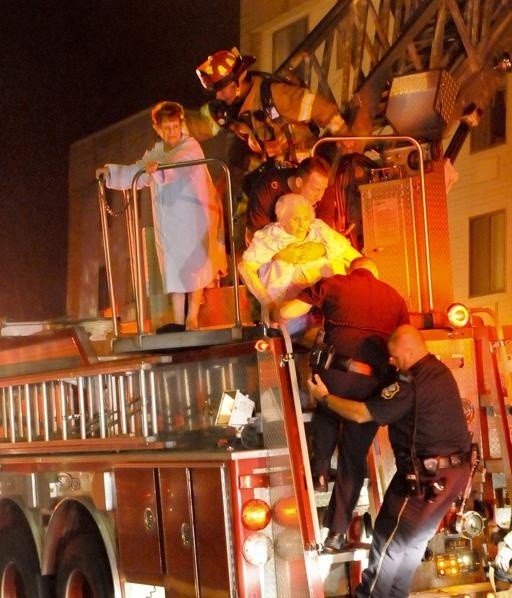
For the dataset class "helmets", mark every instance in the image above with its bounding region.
[196,49,240,90]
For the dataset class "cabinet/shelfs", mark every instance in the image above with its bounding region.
[113,467,237,598]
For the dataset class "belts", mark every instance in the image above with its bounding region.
[438,453,466,468]
[333,357,373,376]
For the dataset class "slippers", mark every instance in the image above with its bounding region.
[156,323,184,333]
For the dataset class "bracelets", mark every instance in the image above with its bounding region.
[320,395,330,408]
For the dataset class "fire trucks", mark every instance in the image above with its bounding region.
[0,139,509,596]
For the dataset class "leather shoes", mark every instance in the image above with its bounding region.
[324,531,344,549]
[314,474,329,491]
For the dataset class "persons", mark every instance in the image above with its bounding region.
[168,47,354,192]
[237,156,333,339]
[93,100,230,331]
[305,323,474,598]
[237,194,369,341]
[273,254,411,555]
